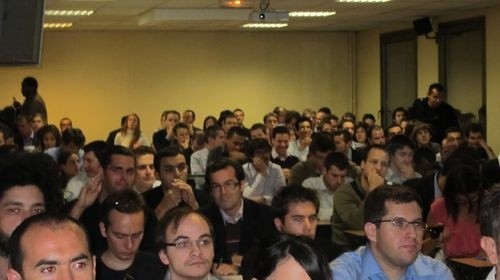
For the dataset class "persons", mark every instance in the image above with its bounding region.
[401,151,462,220]
[92,189,164,280]
[331,145,399,256]
[63,146,142,246]
[269,184,344,271]
[424,146,484,259]
[478,186,500,279]
[0,58,498,201]
[240,233,331,280]
[202,159,271,275]
[0,167,51,280]
[329,185,455,280]
[7,211,96,280]
[139,144,208,230]
[156,204,218,280]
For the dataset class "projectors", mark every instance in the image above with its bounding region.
[242,11,289,28]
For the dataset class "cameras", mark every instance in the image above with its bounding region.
[428,226,444,238]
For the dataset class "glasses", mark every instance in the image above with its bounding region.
[162,238,215,252]
[210,181,239,193]
[284,216,321,224]
[375,219,428,233]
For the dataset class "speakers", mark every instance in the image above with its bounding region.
[413,16,433,36]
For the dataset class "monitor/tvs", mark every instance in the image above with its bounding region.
[0,0,46,68]
